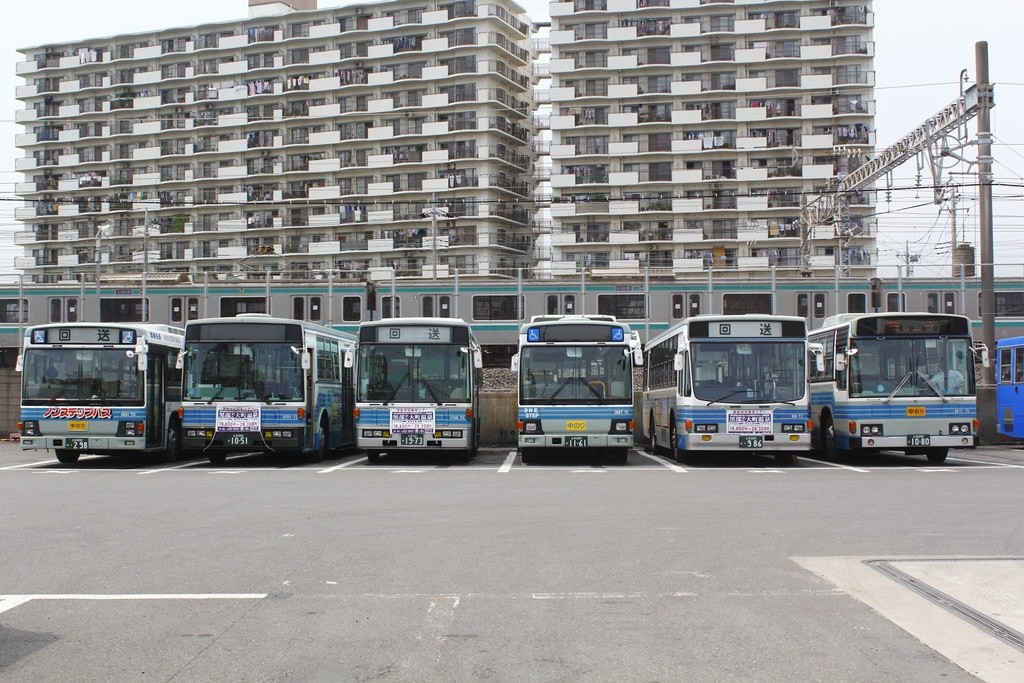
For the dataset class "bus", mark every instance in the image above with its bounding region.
[644,313,825,463]
[807,312,990,462]
[176,312,358,463]
[345,317,483,463]
[510,315,644,465]
[997,336,1024,438]
[16,321,186,462]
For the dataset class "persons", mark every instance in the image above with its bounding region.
[925,357,965,395]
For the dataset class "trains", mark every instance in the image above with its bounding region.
[0,274,1024,367]
